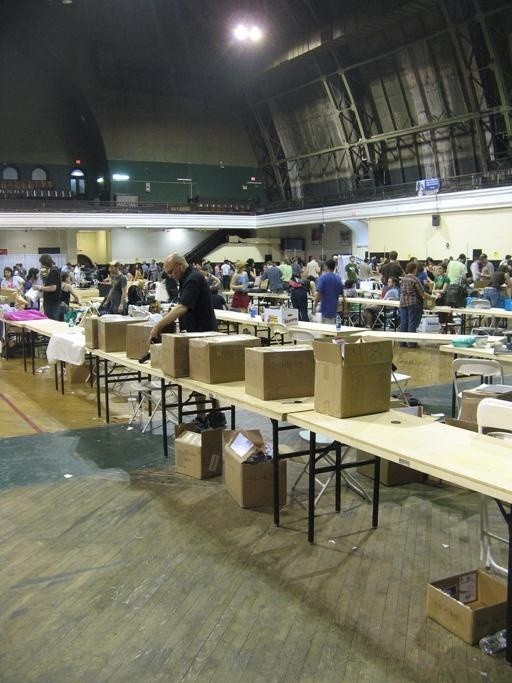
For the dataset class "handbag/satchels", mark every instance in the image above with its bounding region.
[289,280,303,289]
[128,285,142,305]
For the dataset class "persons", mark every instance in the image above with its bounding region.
[147,251,227,430]
[398,264,435,349]
[1,250,512,327]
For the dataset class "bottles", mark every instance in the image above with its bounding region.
[335,311,342,331]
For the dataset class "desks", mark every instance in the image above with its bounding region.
[438,345,512,418]
[227,288,511,334]
[356,326,509,343]
[0,310,84,394]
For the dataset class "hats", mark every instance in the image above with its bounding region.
[236,261,246,271]
[108,261,120,267]
[479,254,487,260]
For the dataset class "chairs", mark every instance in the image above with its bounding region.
[452,358,512,410]
[291,427,371,506]
[391,372,412,405]
[475,394,512,577]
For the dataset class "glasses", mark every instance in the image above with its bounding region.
[166,266,176,275]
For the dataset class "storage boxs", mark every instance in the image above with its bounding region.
[427,391,495,490]
[223,427,289,508]
[426,570,508,646]
[355,450,416,485]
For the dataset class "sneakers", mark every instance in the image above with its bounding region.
[192,412,226,432]
[399,344,420,348]
